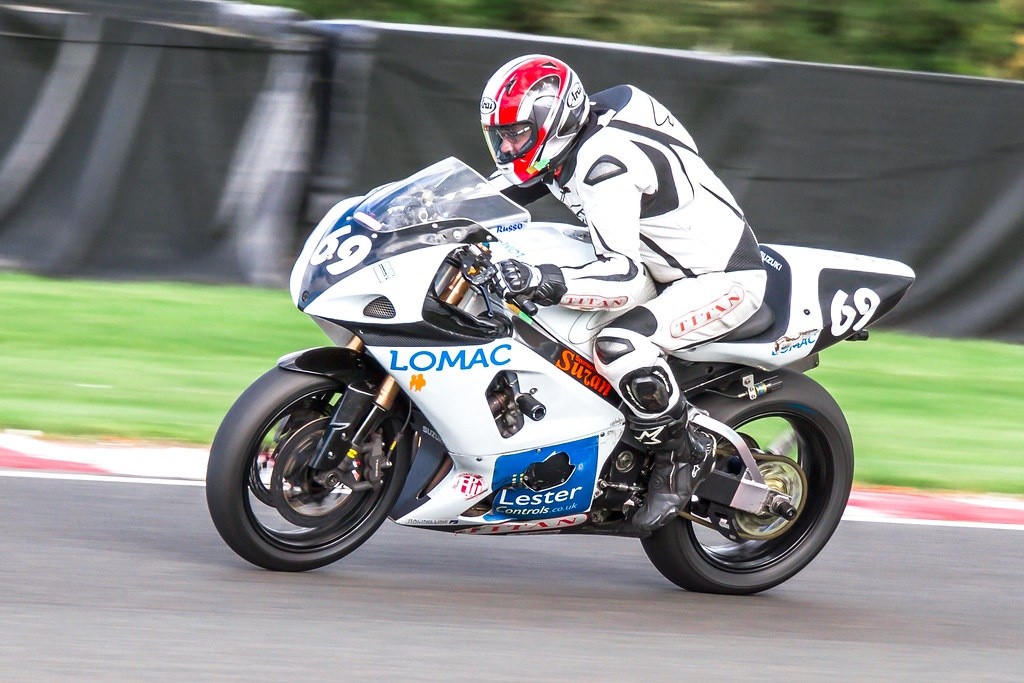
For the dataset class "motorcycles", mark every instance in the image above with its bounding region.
[205,156,915,597]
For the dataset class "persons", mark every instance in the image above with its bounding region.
[387,54,768,531]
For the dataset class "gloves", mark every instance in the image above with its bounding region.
[472,259,568,306]
[387,188,451,229]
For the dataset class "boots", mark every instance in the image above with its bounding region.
[628,390,716,533]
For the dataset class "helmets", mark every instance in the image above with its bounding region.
[480,53,590,188]
